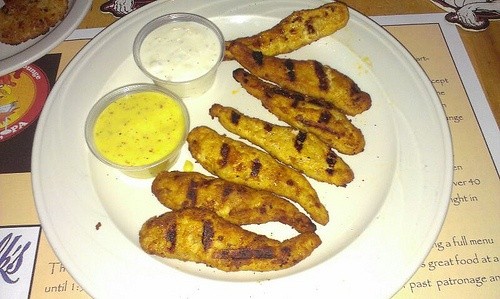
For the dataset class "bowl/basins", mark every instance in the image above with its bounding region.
[85,84,190,178]
[131,13,225,97]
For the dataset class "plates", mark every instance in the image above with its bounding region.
[0,0,92,77]
[31,0,454,299]
